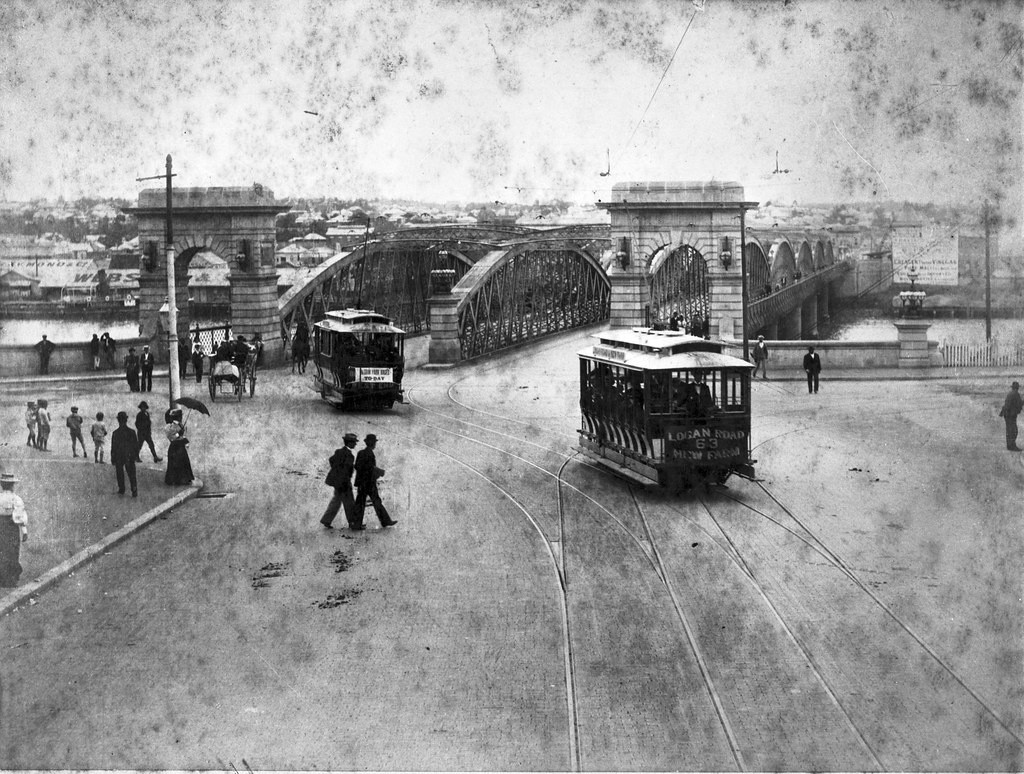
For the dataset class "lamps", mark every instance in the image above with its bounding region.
[141,249,154,272]
[617,248,627,271]
[235,252,248,272]
[720,248,731,271]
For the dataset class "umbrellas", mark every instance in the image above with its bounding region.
[172,397,211,429]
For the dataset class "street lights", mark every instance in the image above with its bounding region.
[898,260,926,320]
[165,154,186,409]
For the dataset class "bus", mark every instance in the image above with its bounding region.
[309,307,409,413]
[572,327,766,492]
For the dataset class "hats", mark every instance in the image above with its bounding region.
[363,435,378,442]
[0,473,20,482]
[758,335,764,339]
[342,433,359,441]
[116,412,128,418]
[170,408,183,416]
[70,406,78,410]
[138,401,149,408]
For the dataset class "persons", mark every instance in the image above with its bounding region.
[0,473,28,588]
[91,413,107,463]
[25,399,51,451]
[66,407,87,458]
[295,320,309,342]
[670,311,710,340]
[803,347,821,393]
[91,332,153,392]
[764,270,803,296]
[179,333,263,397]
[339,334,400,363]
[588,363,714,426]
[111,411,139,496]
[135,402,163,463]
[753,335,768,378]
[1003,381,1024,451]
[320,432,398,530]
[164,408,194,485]
[35,335,56,374]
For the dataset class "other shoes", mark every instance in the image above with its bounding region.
[154,456,163,462]
[382,520,397,527]
[137,457,142,462]
[349,523,366,527]
[351,526,366,531]
[321,520,334,528]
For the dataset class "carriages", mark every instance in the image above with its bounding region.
[207,332,266,402]
